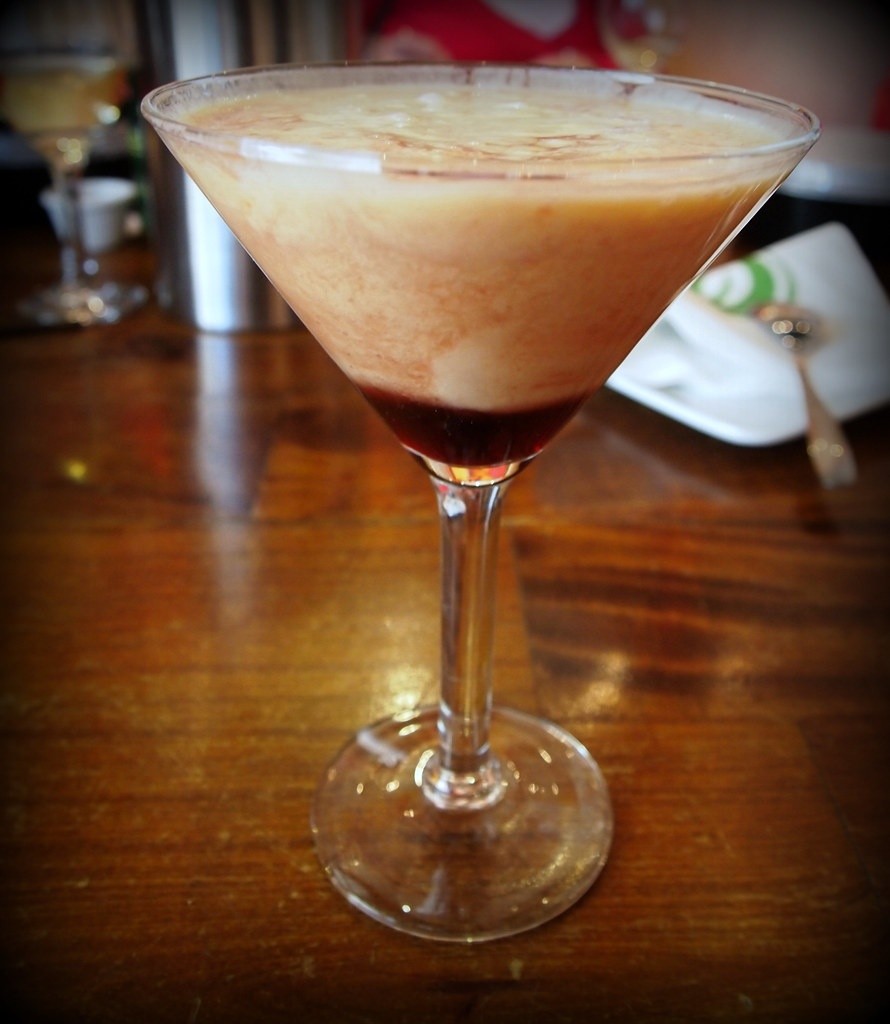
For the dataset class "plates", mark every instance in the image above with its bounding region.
[605,221,890,447]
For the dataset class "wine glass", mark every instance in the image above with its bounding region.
[141,61,820,945]
[1,48,147,324]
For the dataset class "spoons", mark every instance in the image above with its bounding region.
[758,304,855,488]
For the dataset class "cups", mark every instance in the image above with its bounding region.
[40,177,143,255]
